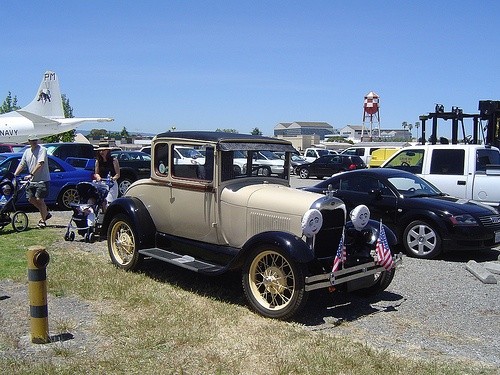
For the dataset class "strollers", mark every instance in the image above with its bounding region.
[0,176,35,232]
[64,178,114,243]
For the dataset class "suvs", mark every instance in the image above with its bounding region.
[0,142,27,153]
[16,143,95,167]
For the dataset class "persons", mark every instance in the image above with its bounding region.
[14,133,52,229]
[0,185,12,212]
[93,140,120,212]
[87,197,94,205]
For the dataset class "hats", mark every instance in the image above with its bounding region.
[28,135,39,140]
[2,185,11,192]
[94,141,112,150]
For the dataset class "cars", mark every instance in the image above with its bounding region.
[100,130,397,320]
[95,145,424,199]
[296,167,500,259]
[295,155,368,179]
[0,153,96,213]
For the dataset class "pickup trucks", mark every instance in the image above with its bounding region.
[378,144,500,212]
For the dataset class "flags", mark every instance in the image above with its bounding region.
[332,229,346,272]
[374,225,393,272]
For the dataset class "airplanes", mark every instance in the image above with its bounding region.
[0,70,114,144]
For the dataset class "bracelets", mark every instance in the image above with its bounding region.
[30,173,34,176]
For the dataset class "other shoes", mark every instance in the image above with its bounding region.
[39,213,51,223]
[37,222,46,229]
